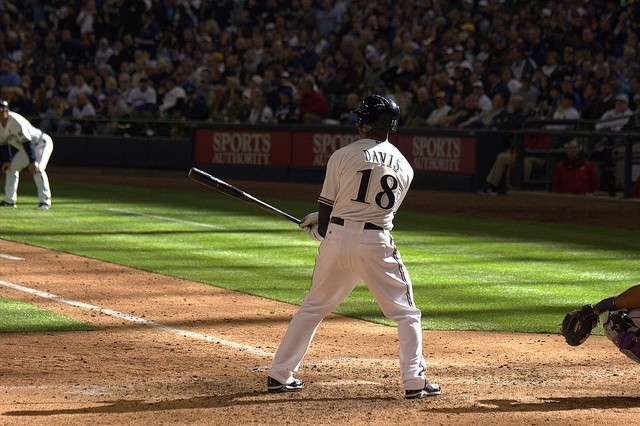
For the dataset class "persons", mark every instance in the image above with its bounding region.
[560,284,640,366]
[267,93,441,402]
[398,93,441,129]
[268,0,639,92]
[55,0,267,137]
[8,100,54,118]
[442,93,640,196]
[0,100,54,211]
[267,92,360,127]
[0,0,54,100]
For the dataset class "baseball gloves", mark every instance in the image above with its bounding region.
[561,304,601,346]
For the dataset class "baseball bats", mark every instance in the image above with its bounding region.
[188,167,314,228]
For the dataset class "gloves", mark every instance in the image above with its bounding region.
[309,223,325,241]
[299,211,319,233]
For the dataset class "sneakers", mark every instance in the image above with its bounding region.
[36,203,50,210]
[404,378,441,399]
[0,201,17,209]
[267,376,304,393]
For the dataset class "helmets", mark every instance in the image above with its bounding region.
[0,100,8,112]
[350,94,400,139]
